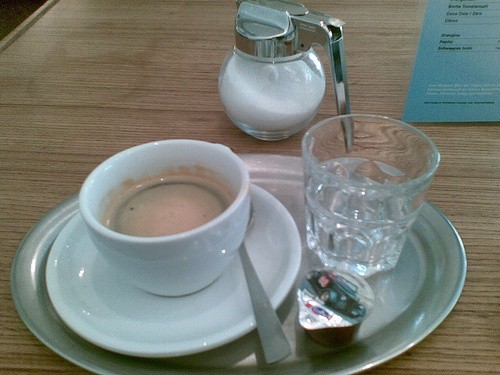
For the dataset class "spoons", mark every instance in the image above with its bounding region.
[238,202,292,366]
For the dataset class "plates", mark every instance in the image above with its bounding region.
[45,184,302,359]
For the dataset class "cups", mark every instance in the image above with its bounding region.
[301,115,441,276]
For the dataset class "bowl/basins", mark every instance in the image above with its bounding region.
[78,138,251,298]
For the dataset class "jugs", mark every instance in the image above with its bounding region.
[218,0,356,155]
